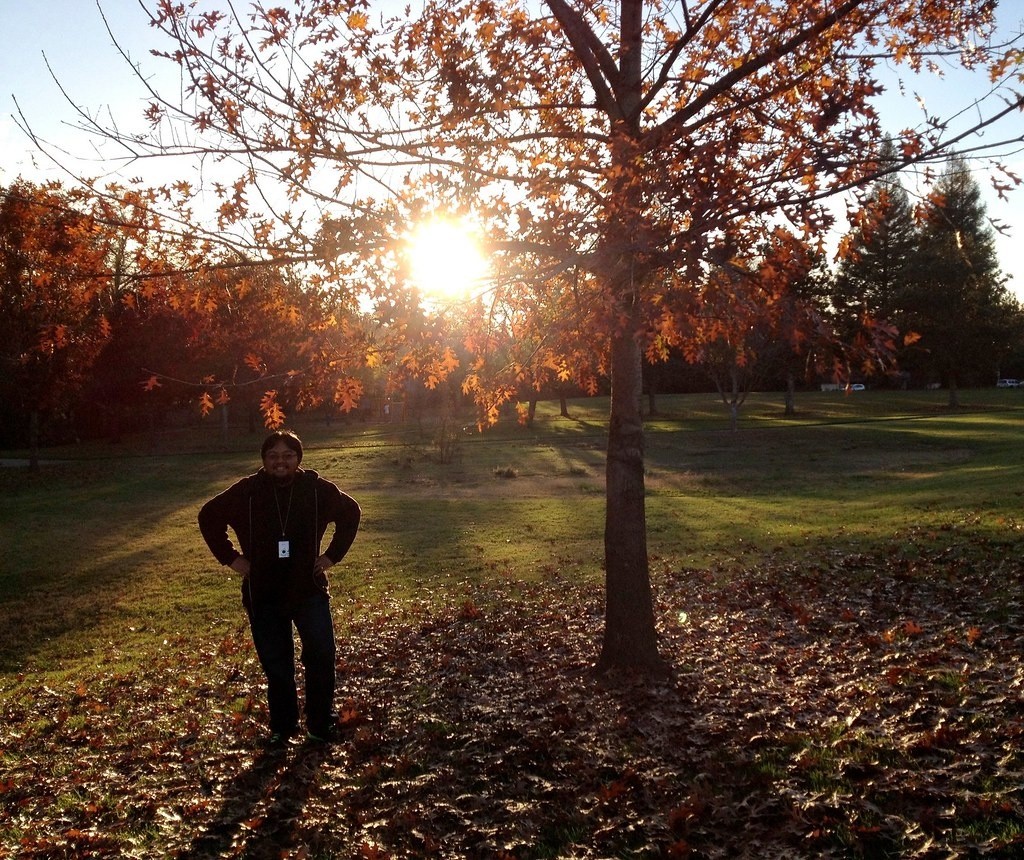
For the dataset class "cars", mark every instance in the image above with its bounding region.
[842,383,865,391]
[997,378,1021,386]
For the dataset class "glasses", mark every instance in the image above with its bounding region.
[264,451,298,461]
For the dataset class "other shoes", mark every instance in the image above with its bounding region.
[265,725,299,754]
[307,711,342,740]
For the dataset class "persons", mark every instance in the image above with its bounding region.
[198,429,361,750]
